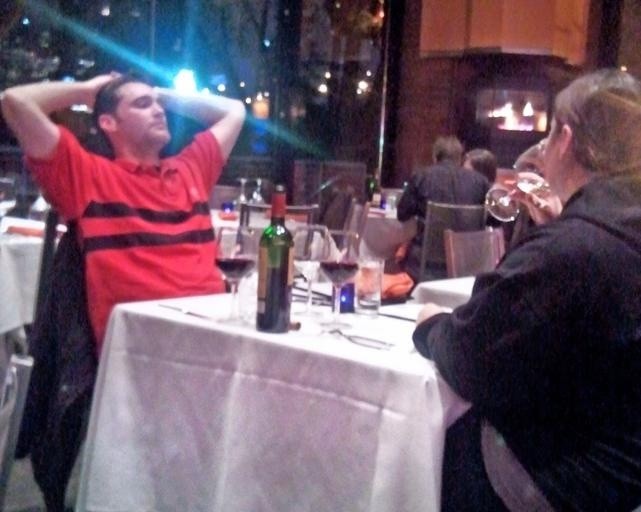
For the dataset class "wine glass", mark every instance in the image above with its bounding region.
[289,224,361,331]
[211,225,256,326]
[481,137,555,224]
[233,176,266,211]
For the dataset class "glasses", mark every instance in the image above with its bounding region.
[484,174,552,223]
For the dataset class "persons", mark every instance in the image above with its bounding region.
[460,145,502,228]
[0,70,249,358]
[408,66,641,512]
[385,133,491,284]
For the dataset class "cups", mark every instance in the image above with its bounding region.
[353,255,383,314]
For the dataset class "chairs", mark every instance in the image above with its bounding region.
[310,169,507,285]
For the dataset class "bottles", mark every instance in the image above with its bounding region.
[253,184,295,335]
